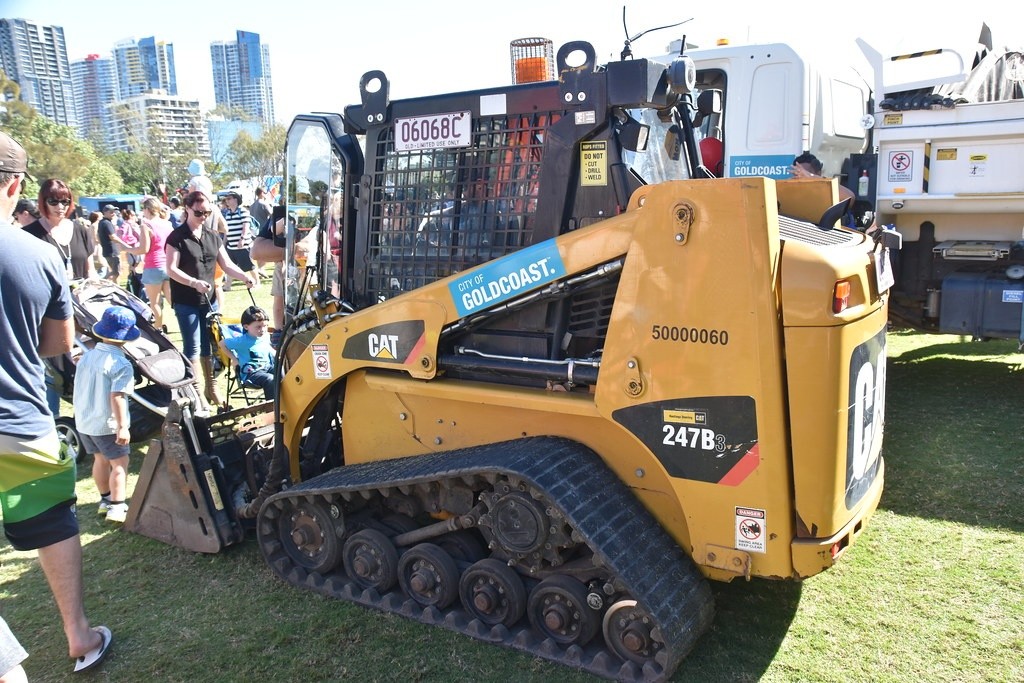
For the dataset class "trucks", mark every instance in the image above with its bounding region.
[600,33,1023,338]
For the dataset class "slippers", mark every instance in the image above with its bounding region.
[70,625,112,675]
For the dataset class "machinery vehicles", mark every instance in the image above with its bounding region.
[117,38,895,683]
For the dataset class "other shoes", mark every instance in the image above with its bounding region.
[98,498,110,514]
[105,506,129,524]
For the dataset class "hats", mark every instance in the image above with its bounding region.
[256,187,267,195]
[101,205,119,213]
[188,159,207,175]
[225,191,242,206]
[0,131,34,182]
[241,306,269,324]
[92,306,141,343]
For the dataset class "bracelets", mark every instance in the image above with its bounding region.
[189,277,196,287]
[240,236,244,239]
[810,172,814,177]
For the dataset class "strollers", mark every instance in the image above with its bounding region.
[44,275,210,463]
[203,281,266,417]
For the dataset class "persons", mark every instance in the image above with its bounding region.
[791,154,856,228]
[0,133,110,672]
[12,157,504,524]
[0,615,30,683]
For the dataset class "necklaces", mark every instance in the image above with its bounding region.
[44,215,73,280]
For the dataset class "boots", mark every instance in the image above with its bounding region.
[200,356,223,406]
[186,360,214,411]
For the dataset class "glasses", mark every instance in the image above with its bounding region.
[45,199,70,207]
[15,174,26,194]
[14,209,29,220]
[188,205,212,217]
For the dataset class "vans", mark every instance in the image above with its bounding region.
[217,179,244,198]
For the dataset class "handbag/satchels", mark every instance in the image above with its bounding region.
[238,208,260,241]
